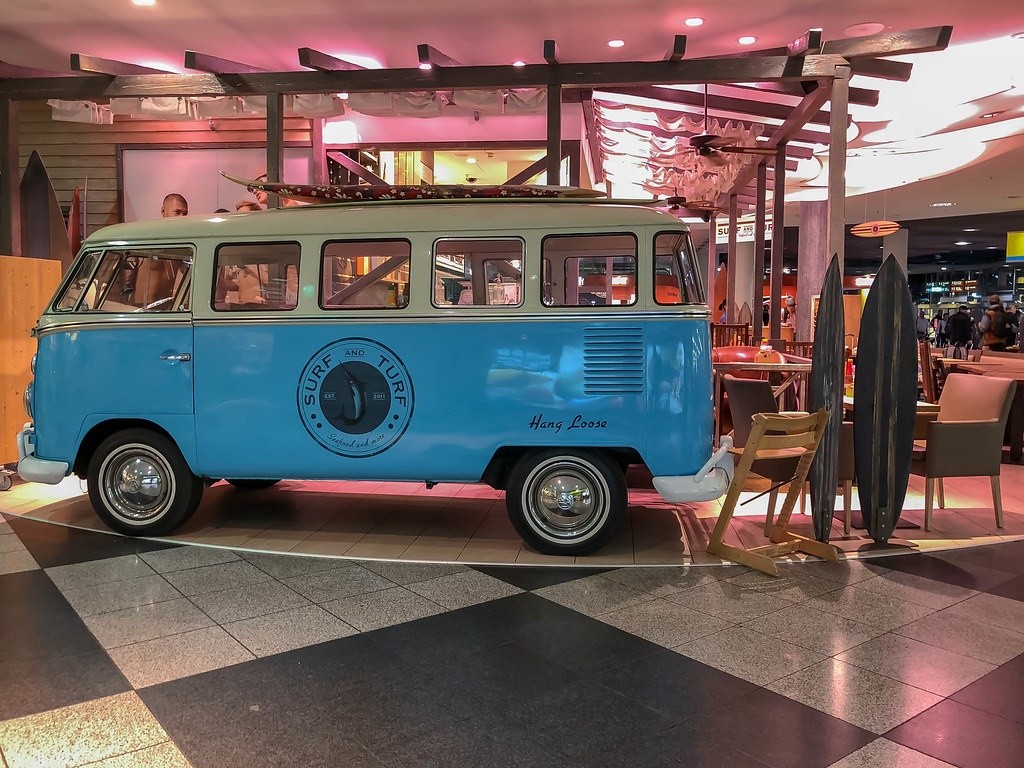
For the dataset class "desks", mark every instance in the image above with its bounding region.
[714,360,813,454]
[832,396,941,530]
[918,353,1024,461]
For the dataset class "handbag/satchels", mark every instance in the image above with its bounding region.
[1012,324,1020,333]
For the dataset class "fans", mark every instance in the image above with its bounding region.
[665,168,723,215]
[667,84,778,166]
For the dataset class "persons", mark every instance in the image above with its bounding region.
[121,174,300,311]
[718,298,796,326]
[916,295,1024,353]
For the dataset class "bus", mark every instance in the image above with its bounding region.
[13,181,737,554]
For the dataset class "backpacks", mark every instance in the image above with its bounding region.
[987,308,1008,338]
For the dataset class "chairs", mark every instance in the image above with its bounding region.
[712,345,856,577]
[911,373,1017,533]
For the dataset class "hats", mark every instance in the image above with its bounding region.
[990,294,1000,305]
[247,174,267,193]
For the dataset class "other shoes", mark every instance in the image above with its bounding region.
[972,346,980,350]
[936,344,939,348]
